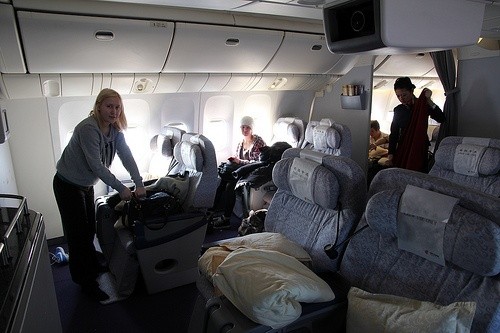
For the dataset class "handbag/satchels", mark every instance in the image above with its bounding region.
[237,210,268,236]
[219,161,238,179]
[122,191,179,230]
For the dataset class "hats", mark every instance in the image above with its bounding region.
[395,77,411,86]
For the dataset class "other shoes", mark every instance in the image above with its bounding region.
[212,215,231,230]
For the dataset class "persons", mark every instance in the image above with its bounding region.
[367,120,389,187]
[52,89,146,302]
[390,77,443,171]
[211,117,266,228]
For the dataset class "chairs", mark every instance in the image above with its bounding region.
[95,116,500,333]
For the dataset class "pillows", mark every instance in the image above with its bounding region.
[219,232,313,264]
[346,286,477,333]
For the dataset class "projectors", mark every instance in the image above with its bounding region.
[321,0,485,57]
